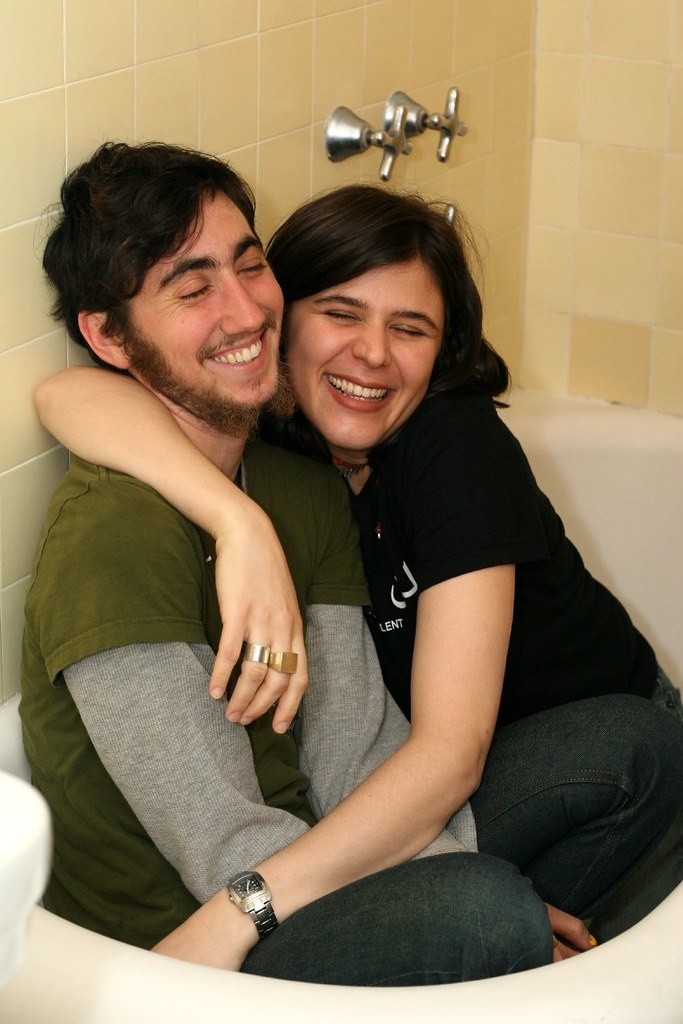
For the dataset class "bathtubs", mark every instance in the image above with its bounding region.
[0,389,683,1024]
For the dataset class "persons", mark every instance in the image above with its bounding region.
[27,186,676,967]
[17,141,683,987]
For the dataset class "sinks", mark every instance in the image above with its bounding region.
[0,770,53,989]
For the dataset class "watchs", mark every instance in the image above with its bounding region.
[225,871,280,941]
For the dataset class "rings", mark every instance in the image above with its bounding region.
[244,643,298,674]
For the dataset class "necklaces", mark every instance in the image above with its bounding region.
[332,454,368,478]
